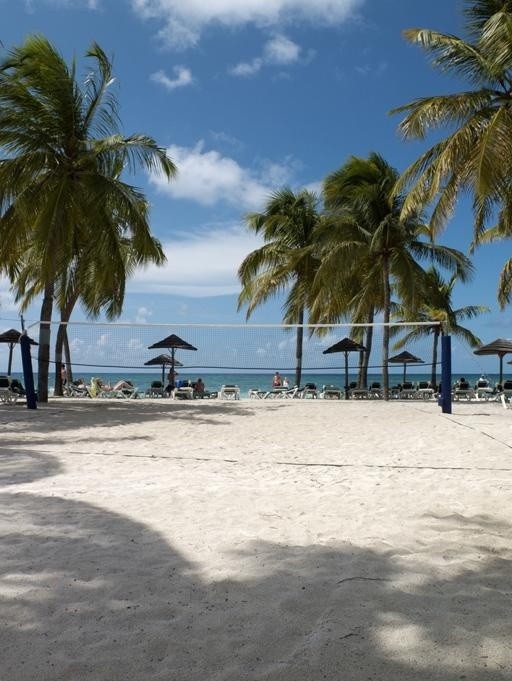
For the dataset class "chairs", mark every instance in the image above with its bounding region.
[1,387,20,405]
[63,381,241,400]
[248,382,442,399]
[451,381,511,403]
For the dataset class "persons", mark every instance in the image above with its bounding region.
[165,368,177,394]
[476,374,490,389]
[187,379,192,387]
[96,377,135,392]
[452,377,470,393]
[60,363,68,390]
[283,377,289,388]
[193,378,205,398]
[273,372,282,387]
